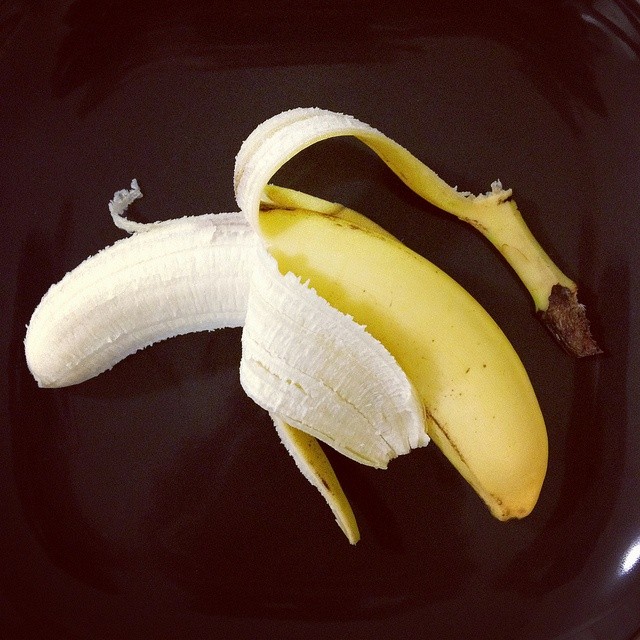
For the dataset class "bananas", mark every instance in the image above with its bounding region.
[24,107,604,546]
